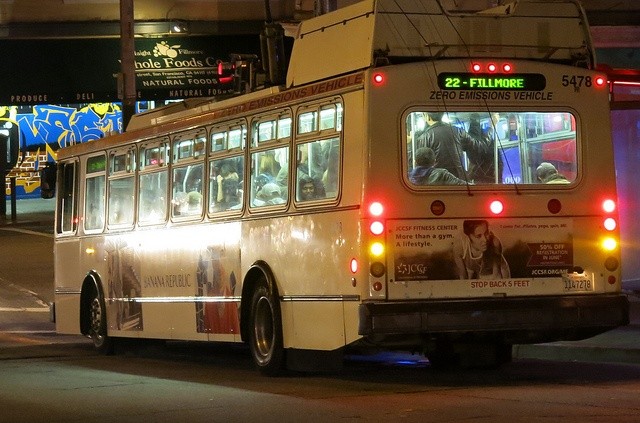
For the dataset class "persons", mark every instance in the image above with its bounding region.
[299,178,323,201]
[200,248,240,334]
[453,220,512,280]
[467,114,504,184]
[407,146,471,186]
[415,110,500,181]
[252,134,339,181]
[257,182,287,205]
[535,160,570,186]
[138,158,240,218]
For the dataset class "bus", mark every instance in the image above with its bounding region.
[50,0,630,377]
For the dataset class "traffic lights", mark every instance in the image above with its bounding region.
[217,57,244,98]
